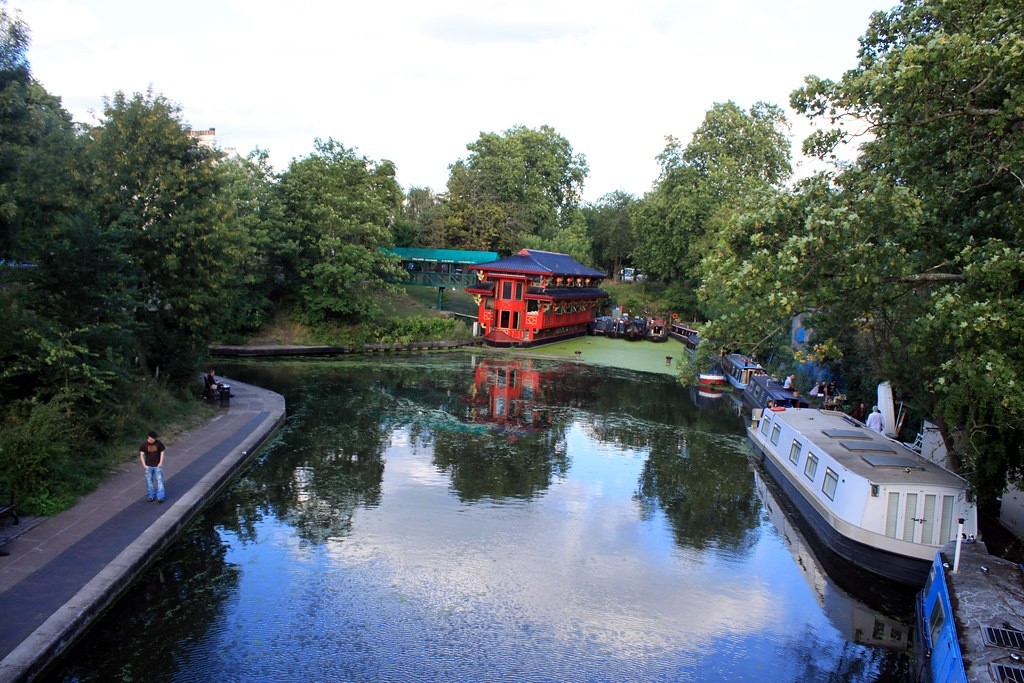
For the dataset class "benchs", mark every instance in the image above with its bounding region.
[204,376,222,404]
[0,491,20,526]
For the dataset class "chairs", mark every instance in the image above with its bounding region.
[903,432,923,451]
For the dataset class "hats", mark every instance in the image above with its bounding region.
[148,431,160,439]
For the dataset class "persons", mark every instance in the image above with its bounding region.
[817,380,845,410]
[866,405,885,433]
[761,400,774,417]
[208,370,235,399]
[854,402,867,426]
[783,372,798,392]
[140,432,166,504]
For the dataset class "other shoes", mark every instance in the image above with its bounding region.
[148,496,155,503]
[157,497,164,503]
[227,393,233,397]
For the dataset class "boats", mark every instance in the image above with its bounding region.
[719,354,766,392]
[593,316,669,342]
[668,321,705,342]
[912,540,1024,683]
[742,376,810,411]
[745,405,978,584]
[683,334,727,385]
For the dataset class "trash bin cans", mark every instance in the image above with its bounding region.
[218,384,232,409]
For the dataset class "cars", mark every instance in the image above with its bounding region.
[620,269,647,284]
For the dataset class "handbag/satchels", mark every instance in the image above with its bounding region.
[211,383,217,389]
